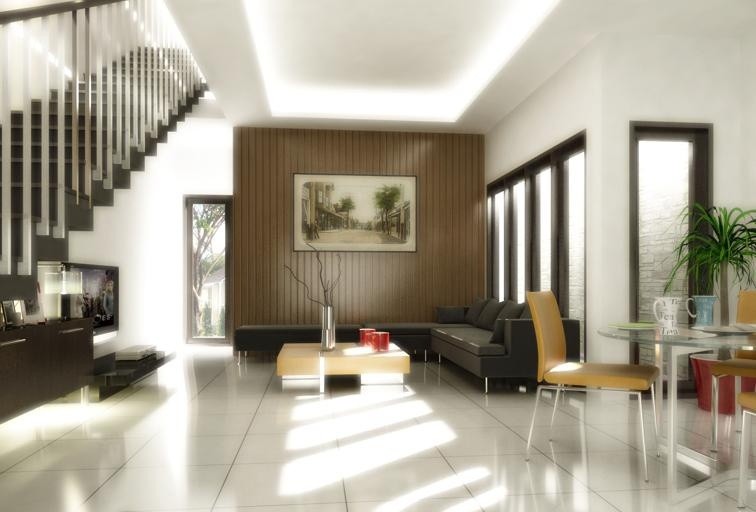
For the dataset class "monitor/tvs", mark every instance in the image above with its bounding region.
[61,261,120,337]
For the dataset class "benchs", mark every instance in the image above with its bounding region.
[235,323,361,358]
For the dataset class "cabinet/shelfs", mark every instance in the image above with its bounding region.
[0,318,91,426]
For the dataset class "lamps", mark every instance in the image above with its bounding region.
[36,261,82,297]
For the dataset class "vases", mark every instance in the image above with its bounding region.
[320,306,334,352]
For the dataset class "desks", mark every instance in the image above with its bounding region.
[596,322,756,502]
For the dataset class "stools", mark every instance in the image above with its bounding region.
[734,390,756,508]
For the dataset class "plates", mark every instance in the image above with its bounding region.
[702,325,754,336]
[606,322,654,333]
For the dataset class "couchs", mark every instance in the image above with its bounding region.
[362,303,581,394]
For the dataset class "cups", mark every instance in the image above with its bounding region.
[653,296,682,336]
[684,295,719,331]
[358,328,377,346]
[370,332,389,353]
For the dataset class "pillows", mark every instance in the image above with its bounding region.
[477,298,504,328]
[489,318,517,344]
[493,299,524,330]
[464,297,488,323]
[436,306,467,323]
[518,302,533,320]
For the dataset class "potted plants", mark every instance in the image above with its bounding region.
[658,201,755,415]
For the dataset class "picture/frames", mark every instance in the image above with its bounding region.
[292,172,417,253]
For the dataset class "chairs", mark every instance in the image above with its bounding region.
[703,289,755,452]
[525,288,661,491]
